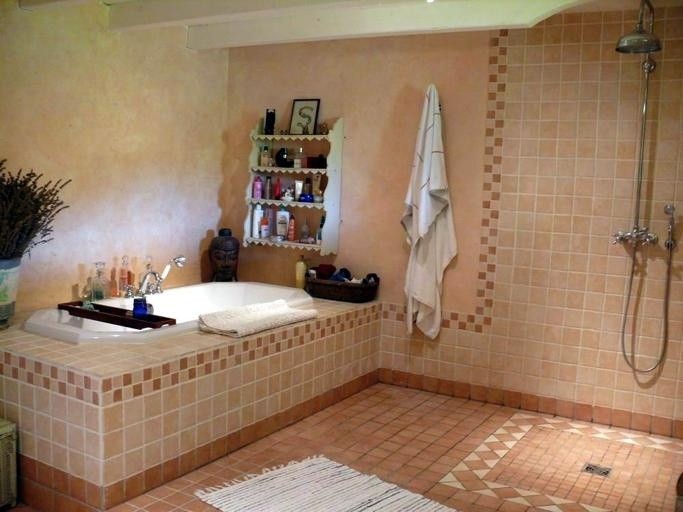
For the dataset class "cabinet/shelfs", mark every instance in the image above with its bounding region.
[241,115,344,256]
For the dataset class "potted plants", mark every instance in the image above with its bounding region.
[0,158,71,331]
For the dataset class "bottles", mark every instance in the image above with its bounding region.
[132,299,148,316]
[295,254,308,287]
[91,257,130,301]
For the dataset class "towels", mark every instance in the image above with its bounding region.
[401,84,458,341]
[198,299,317,338]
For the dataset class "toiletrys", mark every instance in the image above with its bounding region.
[90,255,154,318]
[251,144,327,246]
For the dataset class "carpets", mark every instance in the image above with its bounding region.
[194,453,460,512]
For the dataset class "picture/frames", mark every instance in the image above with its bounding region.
[288,98,320,135]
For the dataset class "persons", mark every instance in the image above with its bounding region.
[206,228,241,283]
